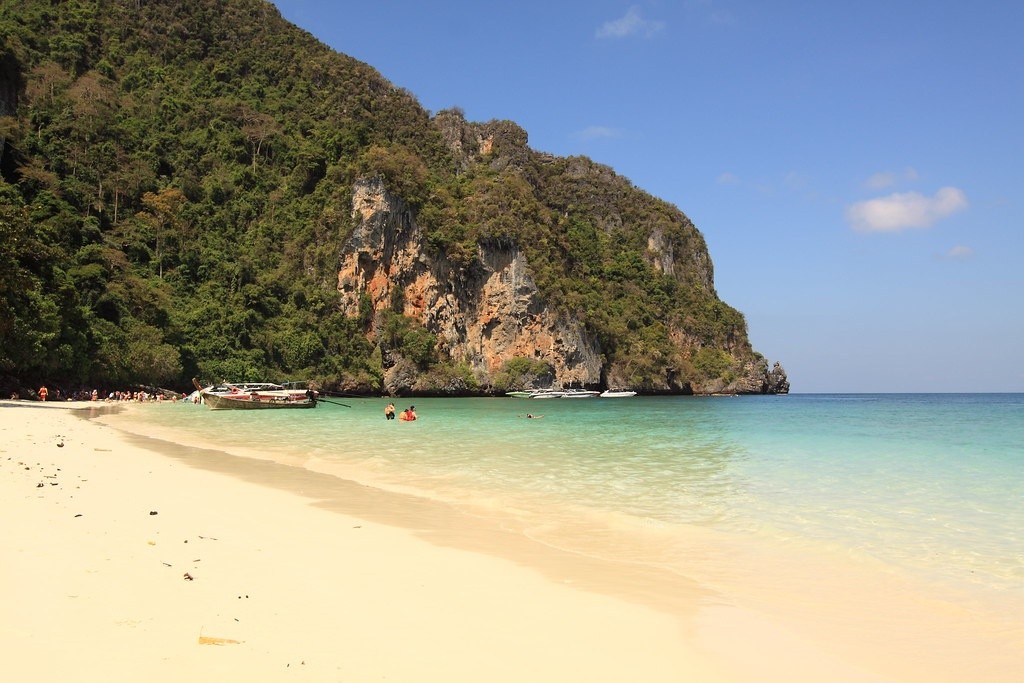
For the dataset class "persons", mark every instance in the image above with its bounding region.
[12,383,199,404]
[385,403,416,421]
[527,414,543,419]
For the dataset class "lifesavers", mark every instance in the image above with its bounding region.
[232,388,238,393]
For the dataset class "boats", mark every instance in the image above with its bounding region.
[505,389,599,399]
[599,389,637,398]
[192,378,318,411]
